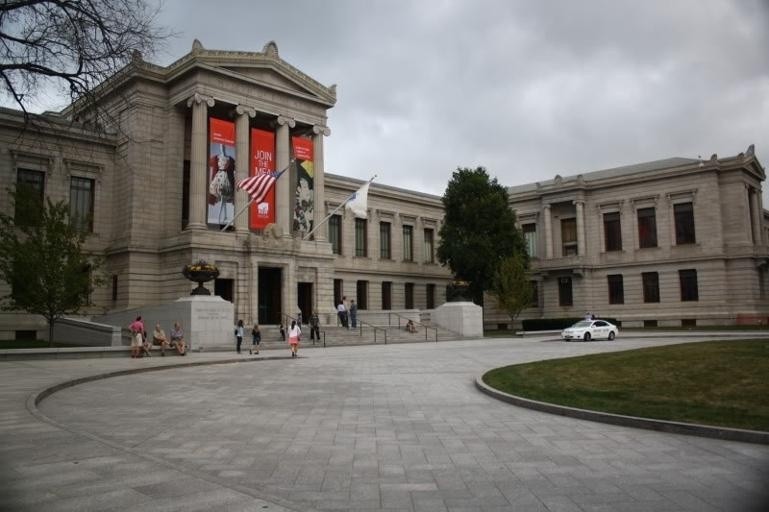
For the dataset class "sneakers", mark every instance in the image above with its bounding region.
[292,352,298,357]
[131,352,186,359]
[249,349,259,354]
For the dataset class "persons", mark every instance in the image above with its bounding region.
[128,316,152,358]
[235,320,243,354]
[250,323,261,354]
[336,301,346,327]
[342,296,348,327]
[349,299,356,328]
[209,143,236,224]
[170,321,185,356]
[407,320,416,333]
[309,310,320,339]
[295,174,313,240]
[153,323,173,356]
[280,314,302,358]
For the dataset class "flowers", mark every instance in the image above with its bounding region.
[450,276,473,290]
[182,258,224,281]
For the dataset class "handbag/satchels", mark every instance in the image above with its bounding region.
[298,337,300,341]
[133,330,138,337]
[235,329,238,335]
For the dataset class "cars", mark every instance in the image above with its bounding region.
[561,319,618,341]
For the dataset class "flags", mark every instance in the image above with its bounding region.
[345,182,368,219]
[236,170,280,204]
